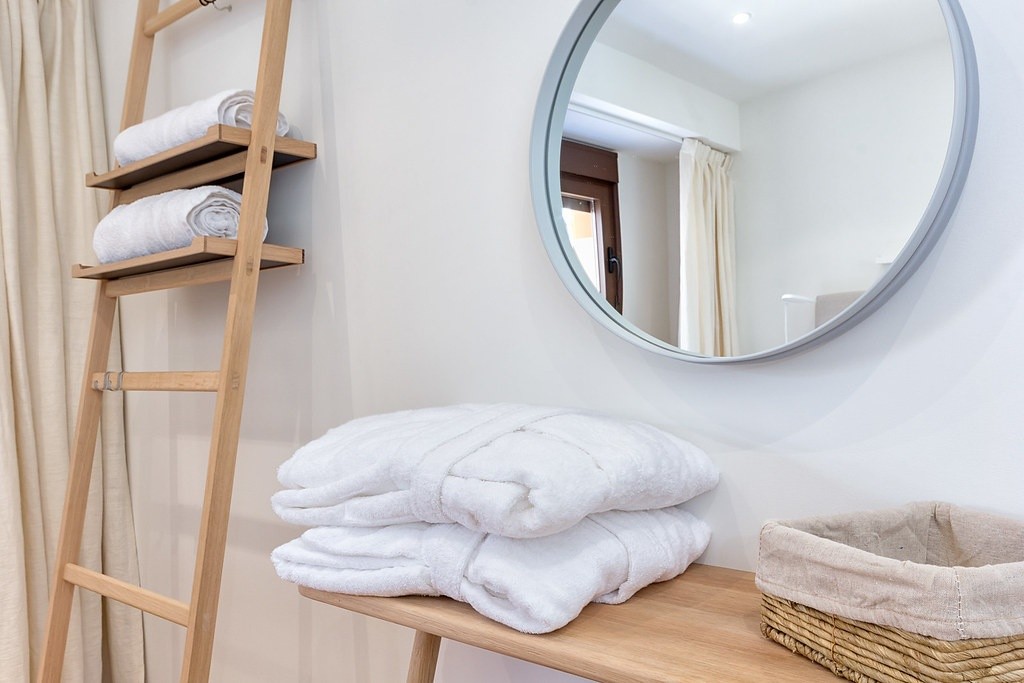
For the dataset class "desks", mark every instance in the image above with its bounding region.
[298,563,855,683]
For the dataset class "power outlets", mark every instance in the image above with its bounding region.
[529,0,980,365]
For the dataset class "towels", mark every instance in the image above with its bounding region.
[91,185,270,265]
[112,88,290,166]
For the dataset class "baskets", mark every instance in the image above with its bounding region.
[756,504,1023,681]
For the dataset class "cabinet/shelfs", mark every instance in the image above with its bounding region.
[70,124,318,270]
